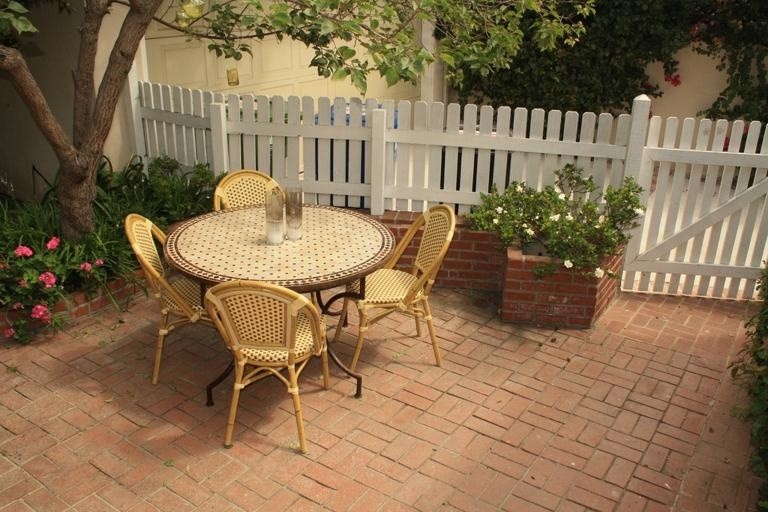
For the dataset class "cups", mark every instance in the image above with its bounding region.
[265,190,285,245]
[285,186,303,241]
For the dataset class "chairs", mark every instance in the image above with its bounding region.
[335,204,456,373]
[124,213,215,386]
[203,280,329,454]
[213,170,285,211]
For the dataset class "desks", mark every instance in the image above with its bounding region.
[163,202,396,406]
